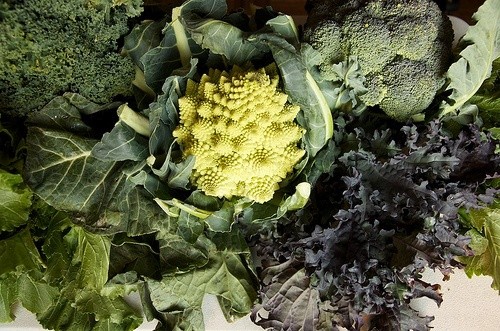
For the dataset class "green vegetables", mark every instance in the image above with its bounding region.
[1,132,268,331]
[255,118,500,331]
[26,0,339,255]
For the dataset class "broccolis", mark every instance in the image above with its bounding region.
[303,0,454,123]
[0,1,145,118]
